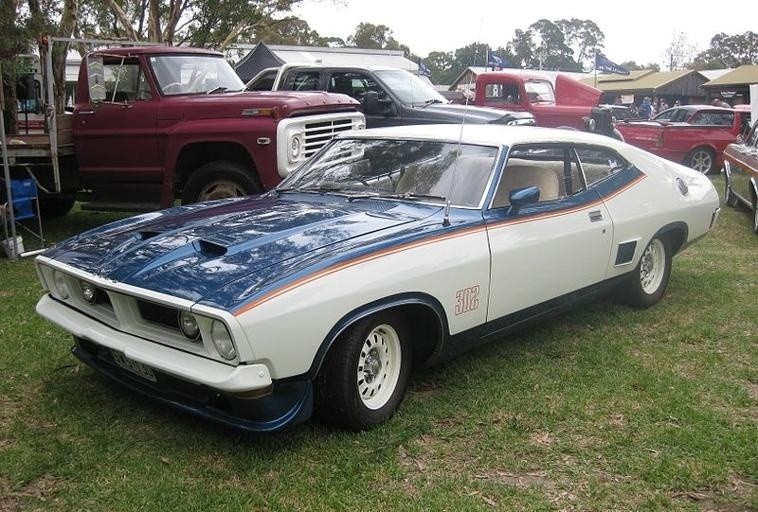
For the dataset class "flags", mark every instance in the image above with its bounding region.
[418,61,433,78]
[596,54,629,75]
[487,49,504,68]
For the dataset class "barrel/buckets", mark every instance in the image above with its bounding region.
[0,235,25,258]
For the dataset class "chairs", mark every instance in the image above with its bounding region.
[0,177,44,263]
[503,84,518,101]
[395,157,609,211]
[104,91,129,103]
[334,76,353,97]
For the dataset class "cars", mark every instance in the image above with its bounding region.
[720,119,758,233]
[612,102,752,175]
[29,120,724,437]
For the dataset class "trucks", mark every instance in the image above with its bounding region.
[0,46,367,219]
[440,73,625,143]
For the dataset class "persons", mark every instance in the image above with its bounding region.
[641,97,669,120]
[712,98,731,110]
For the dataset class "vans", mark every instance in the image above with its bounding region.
[246,63,536,131]
